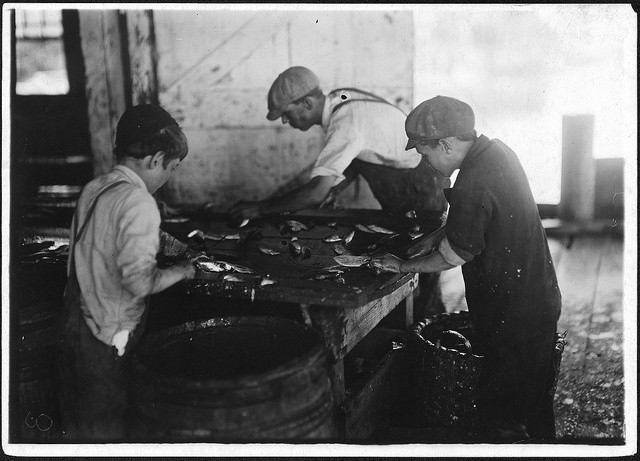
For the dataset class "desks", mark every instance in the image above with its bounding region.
[18,208,420,440]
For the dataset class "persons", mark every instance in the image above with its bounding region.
[227,65,451,313]
[58,104,197,442]
[368,96,563,443]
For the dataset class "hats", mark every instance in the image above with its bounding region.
[266,66,320,120]
[405,96,476,151]
[116,104,175,146]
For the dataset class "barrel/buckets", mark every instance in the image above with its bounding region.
[125,314,330,443]
[411,311,567,442]
